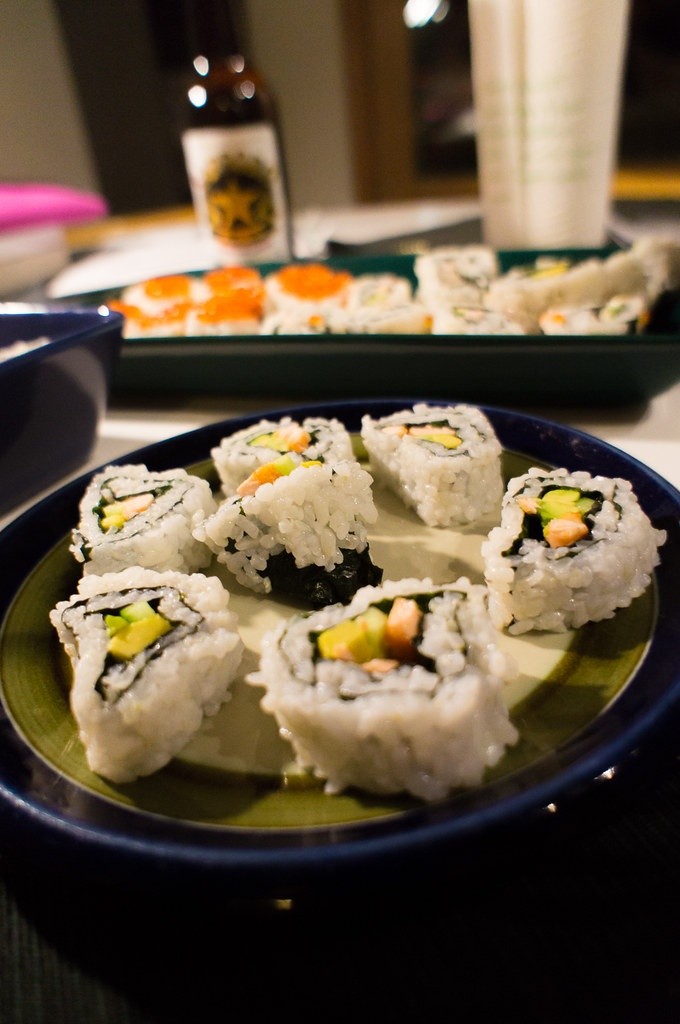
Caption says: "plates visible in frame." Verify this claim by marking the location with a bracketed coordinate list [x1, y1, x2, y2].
[0, 395, 680, 865]
[56, 247, 680, 424]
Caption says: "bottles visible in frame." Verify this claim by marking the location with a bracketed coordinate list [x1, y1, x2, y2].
[171, 0, 294, 266]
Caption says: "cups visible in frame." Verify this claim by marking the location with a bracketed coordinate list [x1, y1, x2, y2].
[469, 0, 629, 252]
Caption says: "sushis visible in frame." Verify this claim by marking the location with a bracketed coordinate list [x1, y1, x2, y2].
[112, 232, 668, 338]
[48, 402, 666, 799]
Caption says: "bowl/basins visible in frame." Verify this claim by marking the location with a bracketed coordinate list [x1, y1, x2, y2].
[0, 311, 121, 507]
